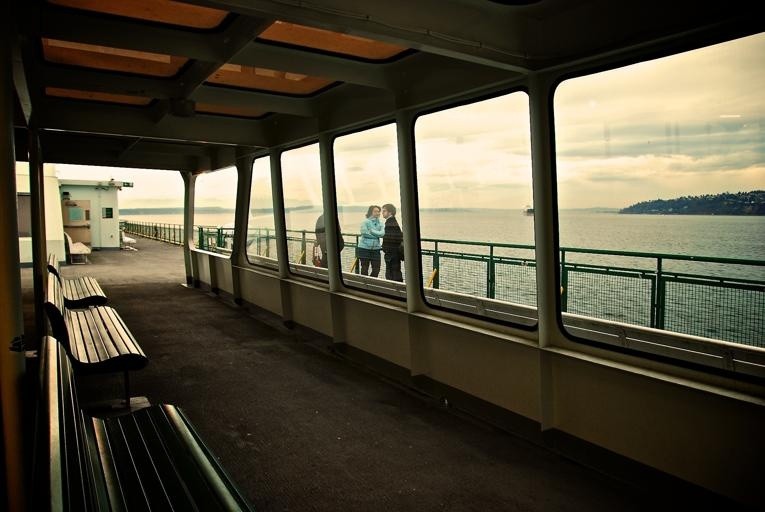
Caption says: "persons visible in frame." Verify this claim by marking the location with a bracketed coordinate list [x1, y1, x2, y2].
[315, 209, 345, 267]
[356, 205, 385, 277]
[381, 203, 404, 282]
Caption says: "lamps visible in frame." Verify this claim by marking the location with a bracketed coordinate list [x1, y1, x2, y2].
[95, 178, 116, 191]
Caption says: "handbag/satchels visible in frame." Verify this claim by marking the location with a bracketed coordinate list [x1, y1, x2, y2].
[391, 230, 404, 262]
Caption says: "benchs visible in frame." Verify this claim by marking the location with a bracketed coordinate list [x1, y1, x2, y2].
[39, 334, 256, 512]
[64, 232, 91, 264]
[45, 272, 152, 418]
[48, 253, 108, 307]
[120, 230, 137, 251]
